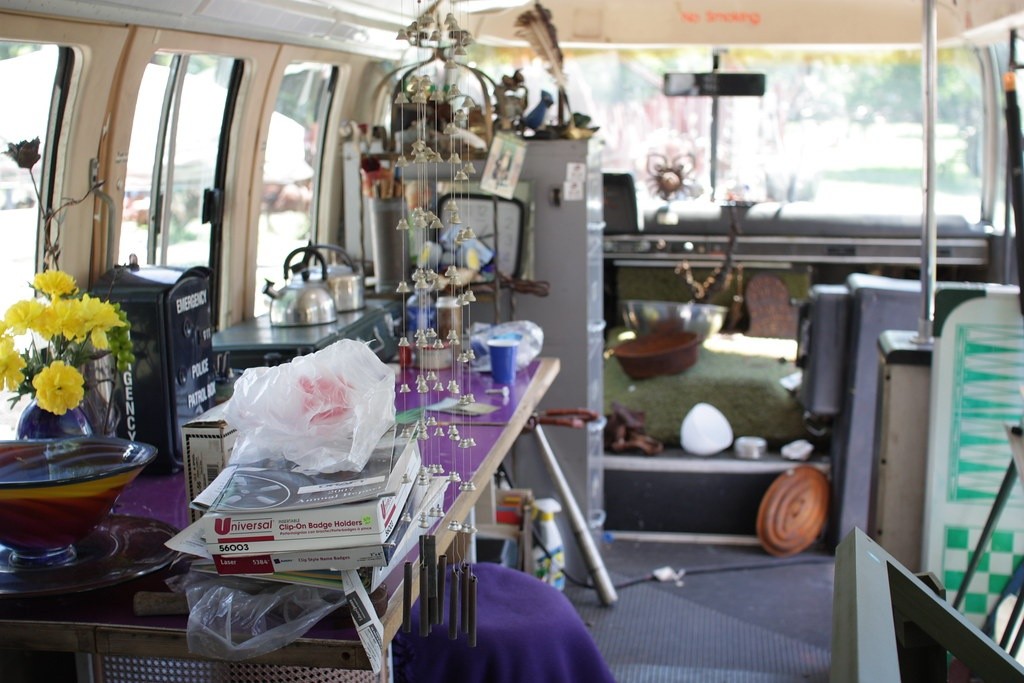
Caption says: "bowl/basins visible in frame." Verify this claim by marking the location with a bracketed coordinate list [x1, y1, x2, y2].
[734, 436, 767, 460]
[622, 299, 727, 344]
[680, 403, 733, 456]
[0, 439, 156, 566]
[613, 333, 699, 378]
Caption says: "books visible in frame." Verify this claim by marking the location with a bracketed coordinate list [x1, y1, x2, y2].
[189, 420, 421, 515]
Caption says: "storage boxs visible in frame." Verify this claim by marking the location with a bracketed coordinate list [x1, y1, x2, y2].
[207, 437, 424, 549]
[212, 466, 432, 576]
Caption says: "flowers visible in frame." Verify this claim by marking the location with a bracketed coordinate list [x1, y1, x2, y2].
[0, 266, 138, 420]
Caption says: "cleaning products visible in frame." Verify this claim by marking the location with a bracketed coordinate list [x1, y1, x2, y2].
[531, 499, 565, 591]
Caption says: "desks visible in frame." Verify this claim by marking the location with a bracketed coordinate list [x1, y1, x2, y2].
[0, 354, 619, 683]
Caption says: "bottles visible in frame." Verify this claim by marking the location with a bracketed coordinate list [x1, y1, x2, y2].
[406, 288, 434, 360]
[435, 284, 461, 359]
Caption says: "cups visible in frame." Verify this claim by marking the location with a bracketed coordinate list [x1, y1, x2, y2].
[487, 340, 519, 384]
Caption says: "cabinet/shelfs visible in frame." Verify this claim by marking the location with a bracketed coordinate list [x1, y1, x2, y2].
[216, 298, 405, 364]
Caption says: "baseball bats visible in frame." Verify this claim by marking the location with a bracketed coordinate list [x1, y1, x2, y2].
[534, 424, 619, 607]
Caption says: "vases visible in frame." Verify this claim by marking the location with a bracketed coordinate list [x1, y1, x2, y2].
[16, 396, 95, 440]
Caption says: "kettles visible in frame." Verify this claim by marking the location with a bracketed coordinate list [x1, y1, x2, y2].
[291, 245, 364, 312]
[262, 247, 336, 325]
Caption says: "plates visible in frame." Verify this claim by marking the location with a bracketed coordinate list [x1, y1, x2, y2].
[0, 515, 180, 598]
